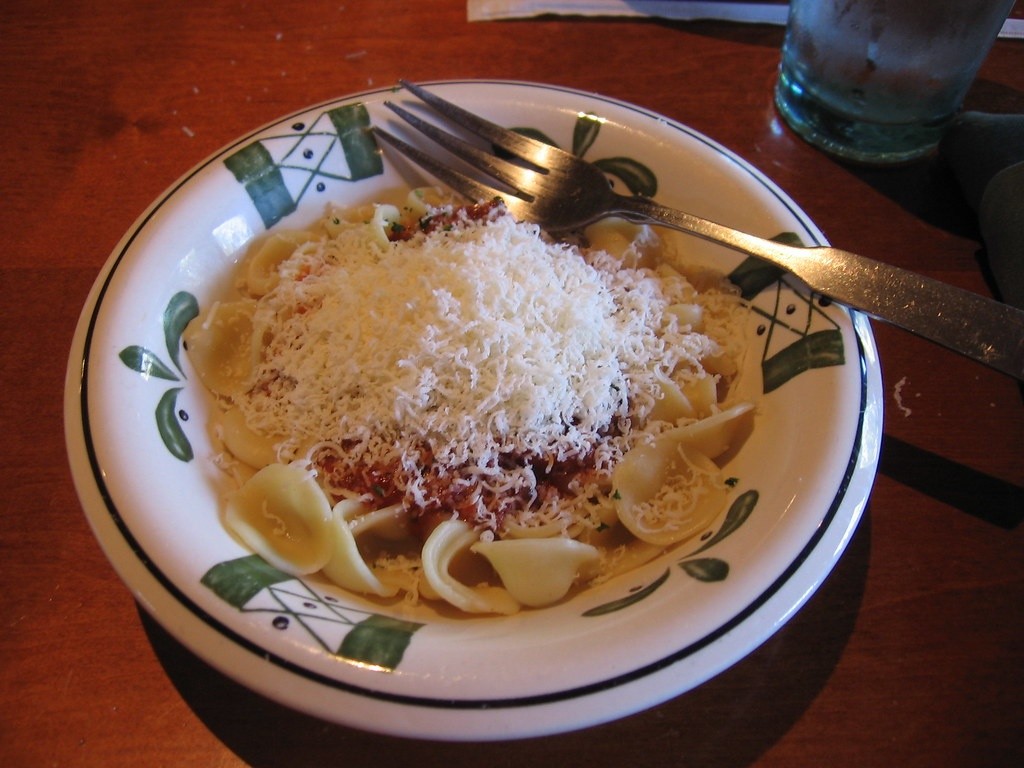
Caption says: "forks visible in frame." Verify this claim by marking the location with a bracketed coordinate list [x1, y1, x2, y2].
[368, 80, 1024, 384]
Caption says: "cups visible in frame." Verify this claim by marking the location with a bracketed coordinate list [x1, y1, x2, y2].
[775, 1, 1017, 167]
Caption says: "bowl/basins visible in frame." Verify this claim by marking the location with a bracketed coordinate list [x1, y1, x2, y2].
[62, 77, 884, 744]
